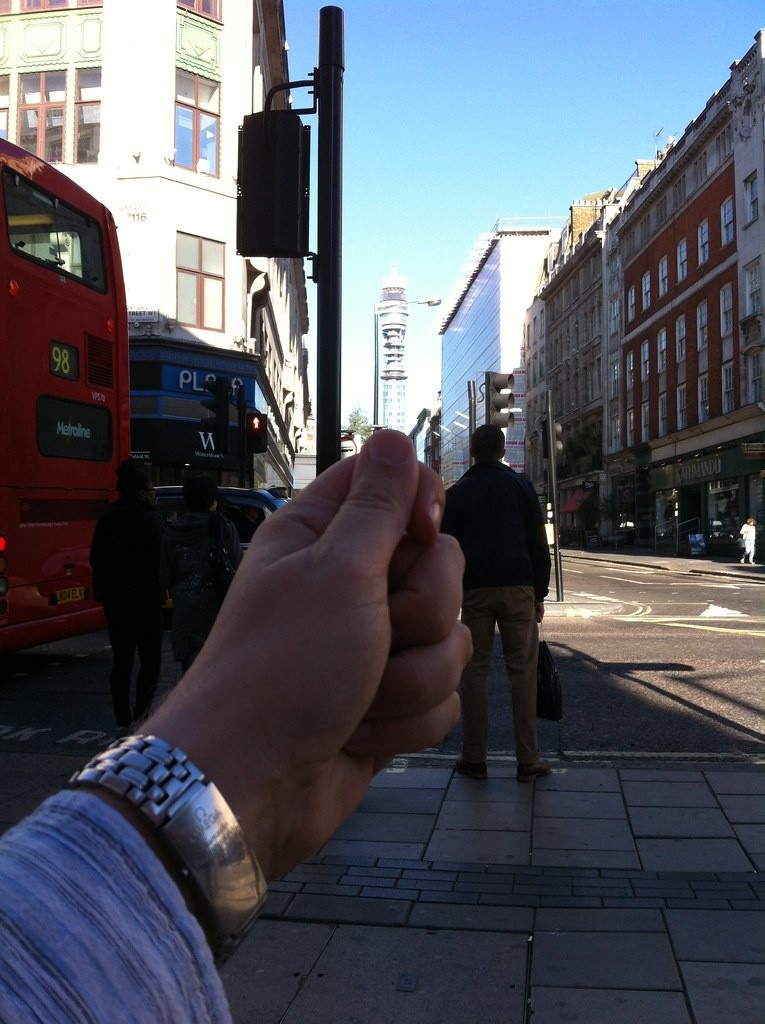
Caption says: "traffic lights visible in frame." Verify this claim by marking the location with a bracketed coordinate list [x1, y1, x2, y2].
[488, 371, 515, 430]
[245, 413, 267, 436]
[552, 419, 563, 455]
[197, 376, 225, 455]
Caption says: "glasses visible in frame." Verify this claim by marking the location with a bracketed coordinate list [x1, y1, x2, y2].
[143, 488, 156, 494]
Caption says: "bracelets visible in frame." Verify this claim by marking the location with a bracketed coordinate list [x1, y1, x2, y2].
[67, 735, 268, 972]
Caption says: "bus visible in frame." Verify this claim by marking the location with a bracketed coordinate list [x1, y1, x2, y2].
[0, 139, 130, 656]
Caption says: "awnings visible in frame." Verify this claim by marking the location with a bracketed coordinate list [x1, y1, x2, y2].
[560, 486, 594, 513]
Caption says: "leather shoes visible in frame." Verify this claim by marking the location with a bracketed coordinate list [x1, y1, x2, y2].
[516, 761, 551, 783]
[456, 758, 487, 779]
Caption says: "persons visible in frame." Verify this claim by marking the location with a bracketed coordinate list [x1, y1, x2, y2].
[439, 425, 552, 783]
[741, 518, 757, 564]
[663, 501, 674, 530]
[90, 459, 259, 737]
[0, 428, 473, 1024]
[715, 490, 739, 529]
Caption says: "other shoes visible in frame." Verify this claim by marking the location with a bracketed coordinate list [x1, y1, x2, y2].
[117, 719, 135, 731]
[132, 707, 149, 723]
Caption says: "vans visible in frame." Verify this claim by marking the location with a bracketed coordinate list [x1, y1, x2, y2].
[149, 485, 288, 561]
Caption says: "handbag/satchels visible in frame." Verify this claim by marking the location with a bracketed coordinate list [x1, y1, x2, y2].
[211, 514, 235, 597]
[535, 640, 564, 722]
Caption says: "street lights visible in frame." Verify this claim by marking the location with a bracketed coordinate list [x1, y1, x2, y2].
[371, 295, 442, 435]
